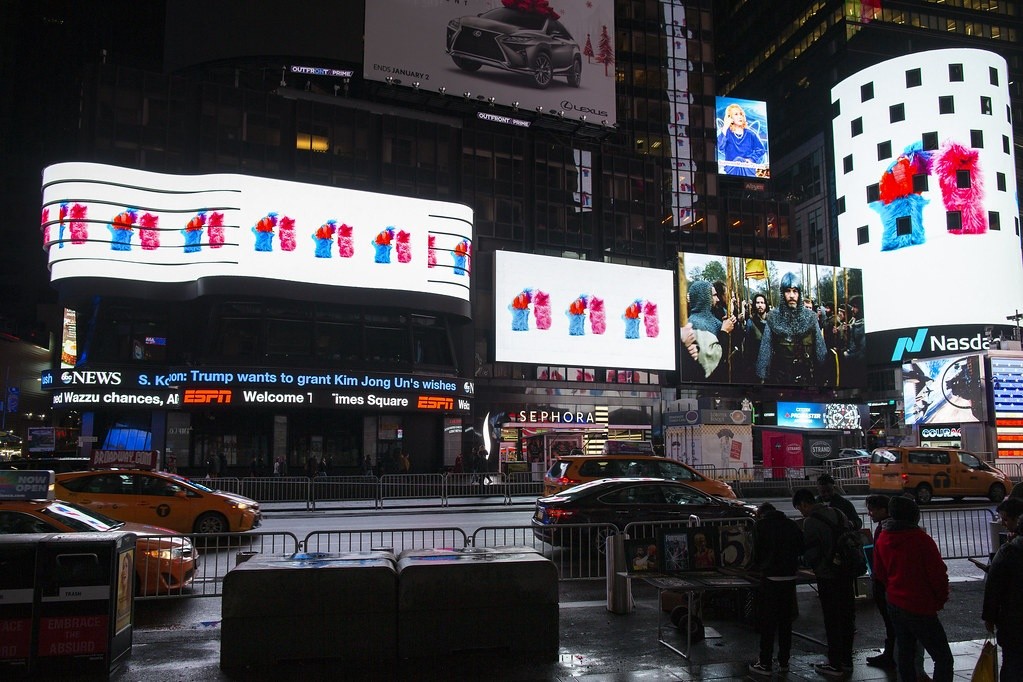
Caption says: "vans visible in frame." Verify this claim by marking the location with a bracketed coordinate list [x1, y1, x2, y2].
[868, 447, 1011, 506]
[544, 455, 737, 501]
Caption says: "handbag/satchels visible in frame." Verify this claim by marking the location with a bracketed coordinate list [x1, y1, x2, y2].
[972, 632, 998, 682]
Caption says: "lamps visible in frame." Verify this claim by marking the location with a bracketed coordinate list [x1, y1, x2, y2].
[601, 120, 608, 127]
[558, 110, 565, 120]
[385, 76, 393, 88]
[580, 115, 587, 124]
[536, 106, 543, 116]
[412, 81, 420, 93]
[438, 86, 446, 97]
[343, 78, 349, 98]
[512, 101, 519, 112]
[488, 97, 496, 109]
[463, 92, 471, 103]
[989, 377, 1001, 390]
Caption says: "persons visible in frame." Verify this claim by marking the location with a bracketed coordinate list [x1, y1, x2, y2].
[717, 105, 765, 177]
[815, 470, 863, 632]
[360, 454, 409, 476]
[680, 280, 768, 382]
[121, 553, 132, 610]
[719, 436, 731, 478]
[866, 495, 894, 666]
[306, 455, 335, 475]
[975, 481, 1023, 682]
[801, 294, 866, 386]
[273, 455, 287, 477]
[873, 497, 954, 682]
[746, 503, 799, 674]
[757, 271, 837, 385]
[792, 488, 854, 676]
[633, 546, 657, 570]
[455, 445, 493, 483]
[250, 456, 264, 480]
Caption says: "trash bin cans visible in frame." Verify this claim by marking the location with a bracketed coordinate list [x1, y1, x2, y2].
[990, 521, 1009, 553]
[833, 466, 854, 477]
[606, 534, 631, 612]
[0, 531, 138, 678]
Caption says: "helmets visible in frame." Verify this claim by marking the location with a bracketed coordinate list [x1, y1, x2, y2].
[780, 274, 806, 315]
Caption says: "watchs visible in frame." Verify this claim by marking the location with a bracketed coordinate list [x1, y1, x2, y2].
[823, 404, 862, 430]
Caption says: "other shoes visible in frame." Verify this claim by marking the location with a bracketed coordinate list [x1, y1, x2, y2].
[866, 655, 897, 667]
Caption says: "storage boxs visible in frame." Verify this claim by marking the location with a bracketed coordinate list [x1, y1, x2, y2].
[660, 590, 683, 612]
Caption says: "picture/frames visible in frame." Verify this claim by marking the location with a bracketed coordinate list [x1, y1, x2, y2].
[655, 527, 688, 572]
[623, 538, 658, 575]
[687, 526, 721, 572]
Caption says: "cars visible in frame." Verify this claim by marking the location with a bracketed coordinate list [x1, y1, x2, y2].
[54, 471, 264, 549]
[445, 6, 582, 90]
[0, 470, 200, 596]
[839, 448, 869, 462]
[531, 478, 760, 560]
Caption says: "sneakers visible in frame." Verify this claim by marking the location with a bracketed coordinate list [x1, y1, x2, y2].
[841, 663, 854, 672]
[813, 664, 844, 676]
[772, 657, 788, 671]
[749, 662, 771, 675]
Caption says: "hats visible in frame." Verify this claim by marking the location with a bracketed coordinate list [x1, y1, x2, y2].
[887, 495, 921, 522]
[849, 295, 863, 309]
[838, 304, 853, 315]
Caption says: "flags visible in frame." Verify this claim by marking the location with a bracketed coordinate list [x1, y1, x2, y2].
[745, 259, 768, 280]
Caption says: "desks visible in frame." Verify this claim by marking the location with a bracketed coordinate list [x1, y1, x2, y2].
[618, 571, 819, 660]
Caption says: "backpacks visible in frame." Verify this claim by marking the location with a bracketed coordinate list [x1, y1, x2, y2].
[811, 508, 867, 572]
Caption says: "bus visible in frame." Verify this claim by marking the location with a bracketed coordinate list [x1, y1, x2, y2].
[27, 427, 80, 455]
